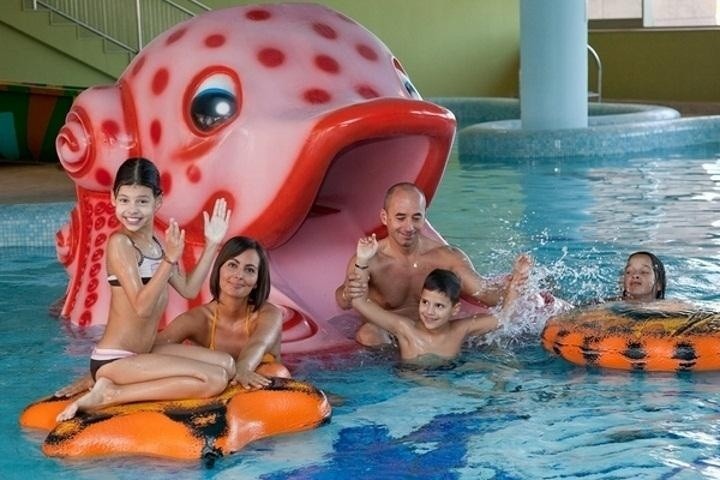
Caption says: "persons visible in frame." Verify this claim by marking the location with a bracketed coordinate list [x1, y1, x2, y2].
[334, 181, 529, 362]
[52, 156, 238, 425]
[53, 235, 292, 400]
[350, 232, 534, 414]
[587, 251, 667, 305]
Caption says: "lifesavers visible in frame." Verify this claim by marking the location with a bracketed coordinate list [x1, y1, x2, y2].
[20, 361, 332, 467]
[541, 300, 719, 373]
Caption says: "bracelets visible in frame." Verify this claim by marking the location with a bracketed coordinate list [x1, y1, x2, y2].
[163, 256, 177, 265]
[355, 263, 368, 269]
